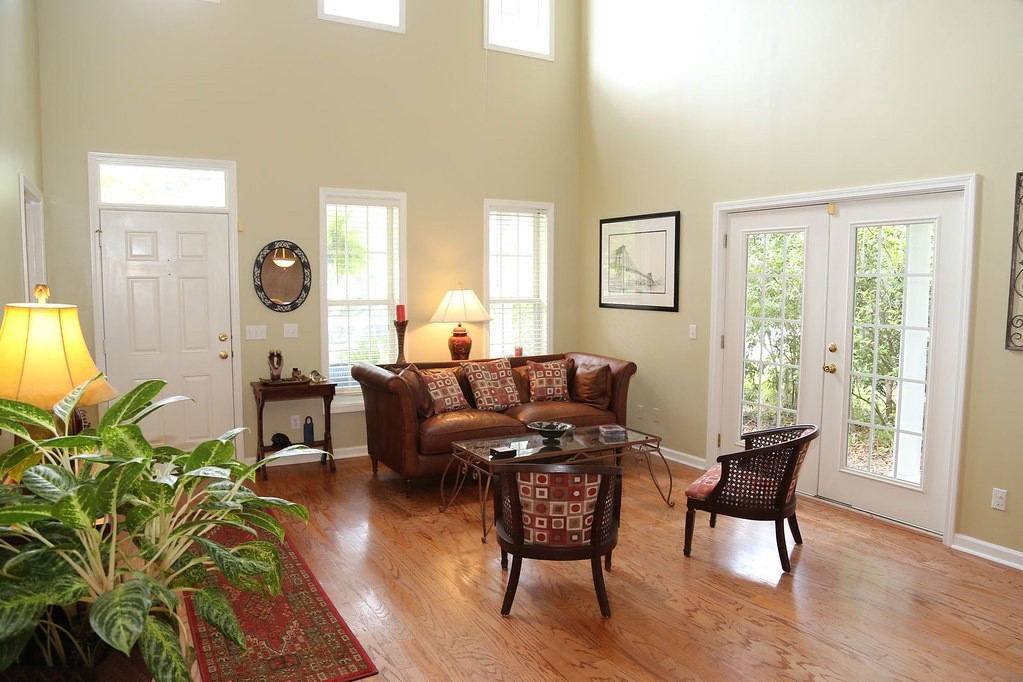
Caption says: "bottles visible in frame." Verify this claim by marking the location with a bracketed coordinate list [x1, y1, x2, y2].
[304, 416, 314, 447]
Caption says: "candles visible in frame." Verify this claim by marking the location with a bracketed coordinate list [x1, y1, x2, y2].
[395, 305, 405, 322]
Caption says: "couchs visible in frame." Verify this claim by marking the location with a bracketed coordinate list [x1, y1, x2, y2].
[351, 351, 638, 498]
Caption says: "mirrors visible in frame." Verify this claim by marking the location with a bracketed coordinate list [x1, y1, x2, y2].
[253, 241, 311, 312]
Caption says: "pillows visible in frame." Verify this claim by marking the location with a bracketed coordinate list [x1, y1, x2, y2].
[527, 359, 572, 403]
[571, 362, 612, 411]
[418, 367, 472, 414]
[459, 357, 522, 411]
[399, 362, 433, 425]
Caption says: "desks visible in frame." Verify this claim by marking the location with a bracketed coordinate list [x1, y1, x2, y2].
[250, 379, 338, 482]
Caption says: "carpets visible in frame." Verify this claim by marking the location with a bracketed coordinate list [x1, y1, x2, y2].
[166, 506, 379, 682]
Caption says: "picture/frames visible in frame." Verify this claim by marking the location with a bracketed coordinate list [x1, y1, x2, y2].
[598, 210, 681, 312]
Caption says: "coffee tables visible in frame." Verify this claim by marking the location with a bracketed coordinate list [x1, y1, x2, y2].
[438, 421, 674, 541]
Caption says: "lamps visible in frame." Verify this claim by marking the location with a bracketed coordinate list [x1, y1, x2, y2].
[429, 282, 493, 361]
[270, 247, 297, 267]
[0, 283, 119, 468]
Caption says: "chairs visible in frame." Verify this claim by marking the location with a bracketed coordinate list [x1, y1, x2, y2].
[683, 424, 820, 570]
[488, 462, 625, 618]
